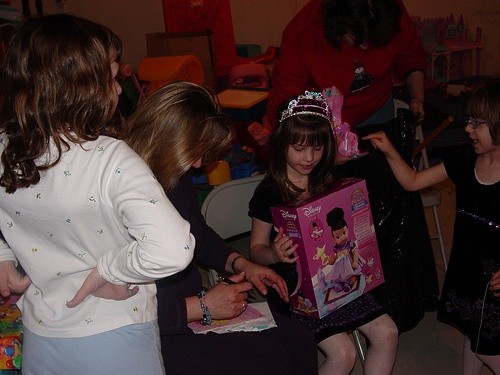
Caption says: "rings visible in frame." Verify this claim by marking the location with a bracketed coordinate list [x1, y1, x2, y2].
[241, 302, 245, 310]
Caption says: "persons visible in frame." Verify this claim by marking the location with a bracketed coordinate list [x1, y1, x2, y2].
[266, 0, 426, 134]
[363, 82, 500, 375]
[118, 82, 318, 374]
[248, 91, 399, 375]
[0, 12, 195, 375]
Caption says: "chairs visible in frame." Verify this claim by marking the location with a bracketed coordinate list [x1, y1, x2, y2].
[202, 175, 367, 366]
[394, 99, 447, 274]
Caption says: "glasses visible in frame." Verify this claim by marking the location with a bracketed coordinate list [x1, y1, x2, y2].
[466, 118, 489, 129]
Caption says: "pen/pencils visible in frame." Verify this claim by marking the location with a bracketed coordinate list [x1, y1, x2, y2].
[217, 273, 256, 299]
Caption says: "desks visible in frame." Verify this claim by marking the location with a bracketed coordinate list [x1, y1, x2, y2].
[428, 39, 484, 83]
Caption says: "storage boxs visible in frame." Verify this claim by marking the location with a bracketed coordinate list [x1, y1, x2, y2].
[270, 179, 385, 318]
[147, 32, 214, 69]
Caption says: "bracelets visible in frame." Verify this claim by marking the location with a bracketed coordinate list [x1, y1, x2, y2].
[232, 256, 245, 274]
[201, 290, 211, 325]
[197, 292, 206, 325]
